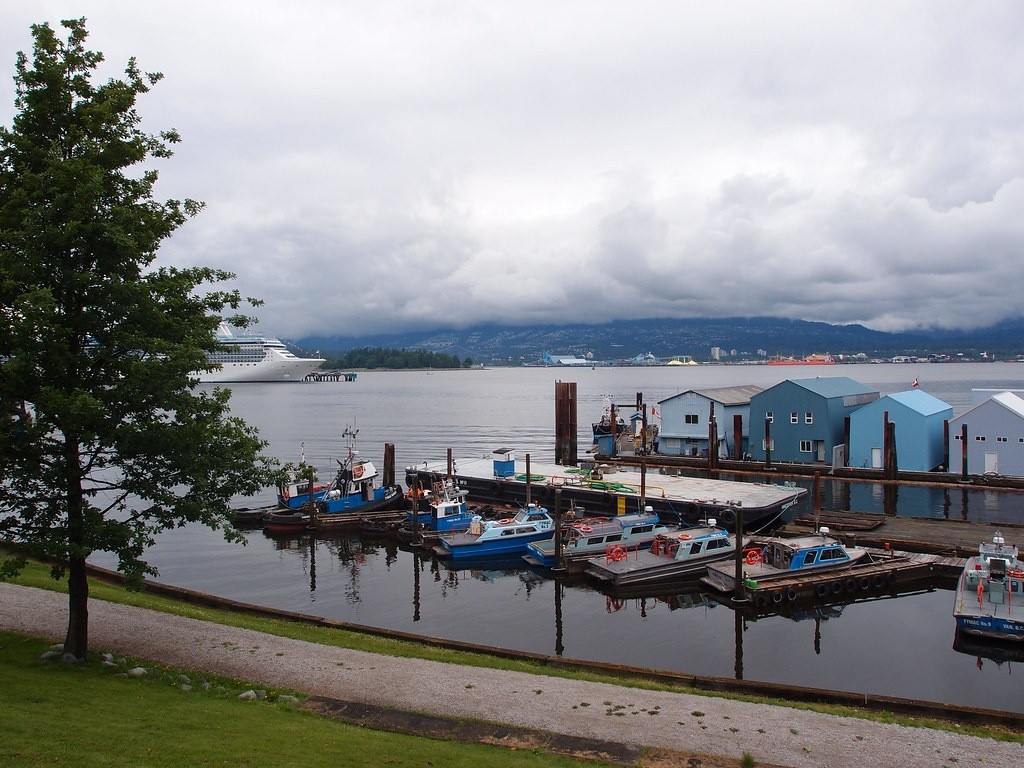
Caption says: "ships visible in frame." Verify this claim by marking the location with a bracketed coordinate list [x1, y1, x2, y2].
[82, 320, 328, 384]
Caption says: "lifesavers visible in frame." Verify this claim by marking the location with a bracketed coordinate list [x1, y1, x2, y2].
[498, 519, 510, 524]
[612, 547, 623, 561]
[354, 466, 363, 477]
[573, 524, 586, 527]
[581, 526, 593, 533]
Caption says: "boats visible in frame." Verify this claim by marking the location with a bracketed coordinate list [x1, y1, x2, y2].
[228, 467, 332, 525]
[952, 623, 1024, 666]
[357, 475, 481, 542]
[262, 459, 404, 531]
[520, 506, 681, 571]
[953, 529, 1024, 639]
[431, 503, 586, 559]
[584, 519, 751, 585]
[699, 526, 867, 593]
[427, 558, 849, 622]
